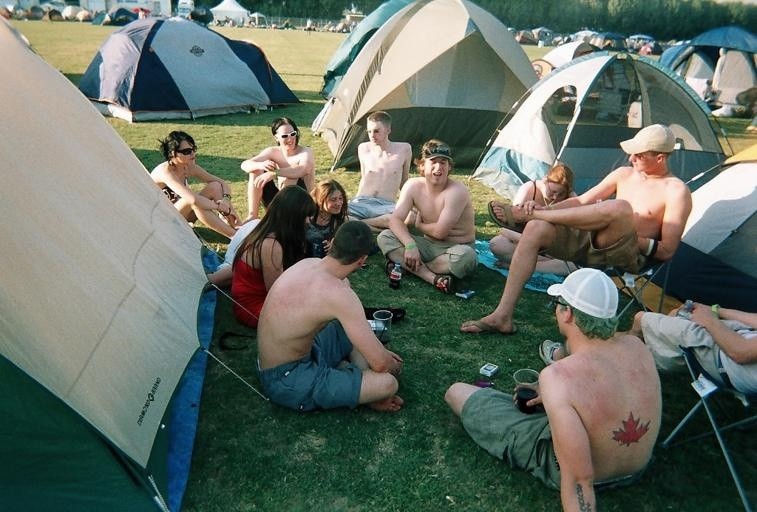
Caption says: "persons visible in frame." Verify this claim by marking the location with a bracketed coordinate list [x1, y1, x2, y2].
[240, 117, 315, 224]
[231, 184, 315, 328]
[346, 111, 413, 231]
[205, 180, 348, 328]
[444, 268, 663, 512]
[149, 130, 242, 239]
[257, 221, 404, 413]
[539, 301, 757, 395]
[376, 139, 477, 294]
[459, 122, 693, 335]
[488, 165, 585, 275]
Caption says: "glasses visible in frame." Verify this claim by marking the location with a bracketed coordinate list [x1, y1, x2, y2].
[274, 131, 298, 139]
[175, 146, 197, 156]
[553, 301, 567, 308]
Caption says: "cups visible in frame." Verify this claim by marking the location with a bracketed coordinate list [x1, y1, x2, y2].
[373, 310, 393, 345]
[513, 369, 540, 415]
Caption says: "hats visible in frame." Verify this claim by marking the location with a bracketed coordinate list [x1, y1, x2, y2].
[422, 155, 454, 162]
[620, 123, 675, 155]
[547, 267, 619, 318]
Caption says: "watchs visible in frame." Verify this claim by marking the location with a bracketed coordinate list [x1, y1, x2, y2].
[223, 193, 231, 199]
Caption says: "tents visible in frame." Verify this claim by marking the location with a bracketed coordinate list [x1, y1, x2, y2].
[317, 0, 426, 100]
[605, 142, 756, 317]
[658, 25, 757, 116]
[77, 18, 305, 121]
[468, 52, 735, 204]
[311, 1, 539, 173]
[0, 17, 269, 512]
[205, 0, 250, 27]
[540, 41, 602, 70]
[513, 26, 666, 56]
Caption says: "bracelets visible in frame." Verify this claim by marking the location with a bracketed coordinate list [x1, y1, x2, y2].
[642, 238, 654, 257]
[274, 169, 279, 178]
[646, 240, 657, 257]
[405, 241, 417, 249]
[711, 304, 720, 318]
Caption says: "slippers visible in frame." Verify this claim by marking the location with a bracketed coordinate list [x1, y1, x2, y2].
[488, 200, 529, 235]
[539, 338, 563, 367]
[461, 319, 519, 335]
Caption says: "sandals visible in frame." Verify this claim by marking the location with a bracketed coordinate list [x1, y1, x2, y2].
[434, 274, 452, 294]
[385, 259, 405, 276]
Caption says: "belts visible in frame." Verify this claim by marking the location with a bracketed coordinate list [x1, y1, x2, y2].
[719, 360, 738, 393]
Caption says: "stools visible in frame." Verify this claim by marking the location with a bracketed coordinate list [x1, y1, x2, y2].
[662, 344, 757, 512]
[562, 259, 670, 328]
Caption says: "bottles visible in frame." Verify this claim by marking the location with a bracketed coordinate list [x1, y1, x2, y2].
[388, 264, 401, 289]
